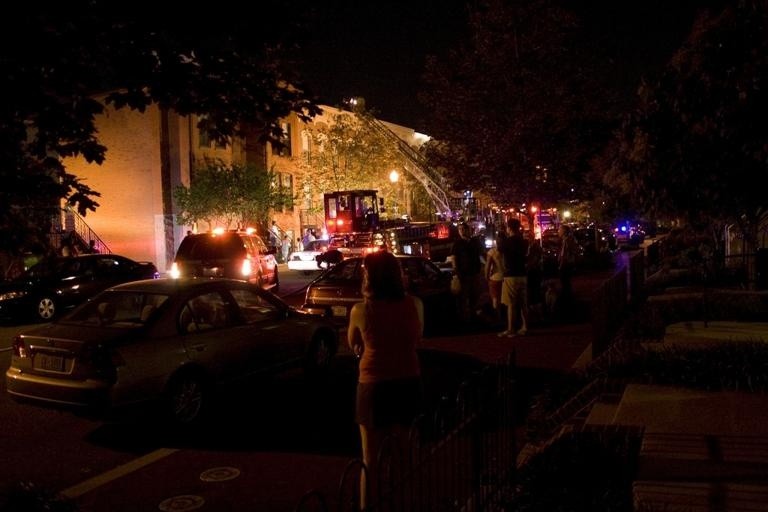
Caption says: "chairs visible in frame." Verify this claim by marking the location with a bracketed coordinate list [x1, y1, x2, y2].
[98, 303, 117, 320]
[141, 305, 157, 320]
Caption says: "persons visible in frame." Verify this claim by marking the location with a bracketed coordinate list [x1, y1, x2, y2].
[83, 240, 99, 254]
[270, 219, 317, 265]
[450, 217, 575, 339]
[364, 207, 377, 232]
[187, 230, 192, 234]
[347, 248, 427, 512]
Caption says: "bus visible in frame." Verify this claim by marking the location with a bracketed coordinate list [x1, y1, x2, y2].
[534, 214, 559, 241]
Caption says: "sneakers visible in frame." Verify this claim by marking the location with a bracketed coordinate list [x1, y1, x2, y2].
[498, 329, 527, 337]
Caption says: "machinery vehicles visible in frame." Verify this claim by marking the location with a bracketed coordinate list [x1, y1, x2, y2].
[313, 190, 486, 272]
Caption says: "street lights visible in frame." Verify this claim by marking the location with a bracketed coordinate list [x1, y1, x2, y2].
[390, 170, 399, 220]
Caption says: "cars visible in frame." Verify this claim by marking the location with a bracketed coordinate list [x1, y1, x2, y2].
[0, 254, 157, 322]
[304, 255, 451, 349]
[5, 275, 341, 425]
[171, 232, 283, 295]
